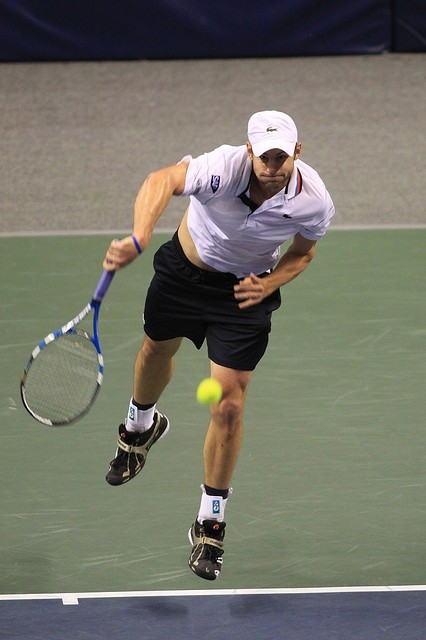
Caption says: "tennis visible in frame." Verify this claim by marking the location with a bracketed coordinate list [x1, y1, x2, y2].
[196, 378, 222, 405]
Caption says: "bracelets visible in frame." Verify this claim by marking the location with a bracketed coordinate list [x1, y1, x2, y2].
[130, 235, 142, 254]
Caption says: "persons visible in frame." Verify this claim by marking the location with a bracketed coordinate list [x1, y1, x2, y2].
[101, 109, 335, 581]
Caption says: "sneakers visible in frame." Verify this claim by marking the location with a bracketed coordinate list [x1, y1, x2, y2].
[106, 410, 170, 485]
[187, 516, 226, 579]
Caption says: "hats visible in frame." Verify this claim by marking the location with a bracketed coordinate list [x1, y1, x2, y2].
[248, 110, 297, 156]
